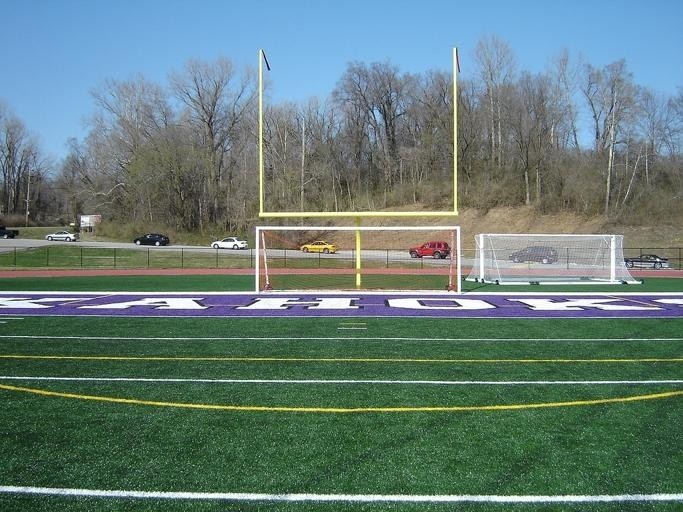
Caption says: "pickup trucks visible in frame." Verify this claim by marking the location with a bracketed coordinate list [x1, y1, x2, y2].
[0, 225, 20, 239]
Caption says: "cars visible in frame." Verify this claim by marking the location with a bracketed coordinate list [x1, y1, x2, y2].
[620, 254, 669, 270]
[45, 230, 80, 241]
[133, 234, 170, 246]
[210, 236, 249, 250]
[300, 240, 337, 254]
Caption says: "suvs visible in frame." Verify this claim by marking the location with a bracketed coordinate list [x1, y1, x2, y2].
[509, 246, 558, 264]
[409, 241, 451, 260]
